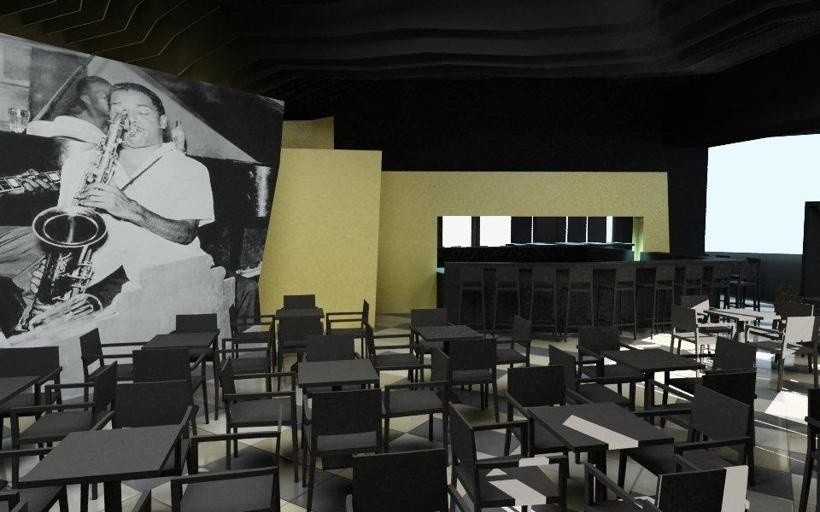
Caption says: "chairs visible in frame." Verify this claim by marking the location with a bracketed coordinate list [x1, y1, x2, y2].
[437, 251, 766, 341]
[0, 294, 819, 512]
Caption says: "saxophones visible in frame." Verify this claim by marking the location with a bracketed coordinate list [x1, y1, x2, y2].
[20, 109, 141, 330]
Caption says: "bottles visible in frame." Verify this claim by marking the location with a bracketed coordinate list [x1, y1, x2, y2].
[173, 121, 186, 156]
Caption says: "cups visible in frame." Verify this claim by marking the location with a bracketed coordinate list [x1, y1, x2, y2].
[8, 106, 31, 134]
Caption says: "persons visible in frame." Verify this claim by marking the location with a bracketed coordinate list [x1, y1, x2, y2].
[64, 76, 112, 146]
[0, 83, 261, 351]
